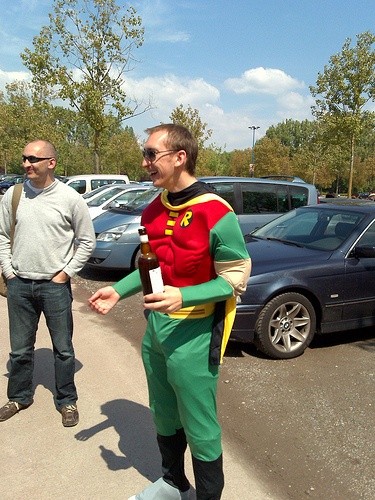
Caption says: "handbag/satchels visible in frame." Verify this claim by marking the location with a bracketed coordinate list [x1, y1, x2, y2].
[0, 183, 23, 298]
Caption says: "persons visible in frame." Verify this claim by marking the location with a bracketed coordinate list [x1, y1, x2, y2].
[89, 125, 252, 500]
[0, 140, 96, 427]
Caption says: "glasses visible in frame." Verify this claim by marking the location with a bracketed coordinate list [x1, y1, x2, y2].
[21, 155, 53, 163]
[141, 148, 179, 162]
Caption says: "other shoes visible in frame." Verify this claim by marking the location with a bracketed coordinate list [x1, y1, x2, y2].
[62, 403, 79, 427]
[1, 401, 23, 421]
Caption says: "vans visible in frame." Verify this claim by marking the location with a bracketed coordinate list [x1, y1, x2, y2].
[84, 172, 319, 273]
[64, 174, 130, 197]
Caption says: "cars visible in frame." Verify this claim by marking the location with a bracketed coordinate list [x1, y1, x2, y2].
[229, 202, 375, 360]
[83, 183, 152, 221]
[0, 174, 25, 194]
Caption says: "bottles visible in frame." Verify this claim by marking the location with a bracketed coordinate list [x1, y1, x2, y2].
[138, 226, 164, 303]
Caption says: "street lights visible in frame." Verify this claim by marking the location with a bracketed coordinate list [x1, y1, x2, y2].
[248, 125, 260, 177]
